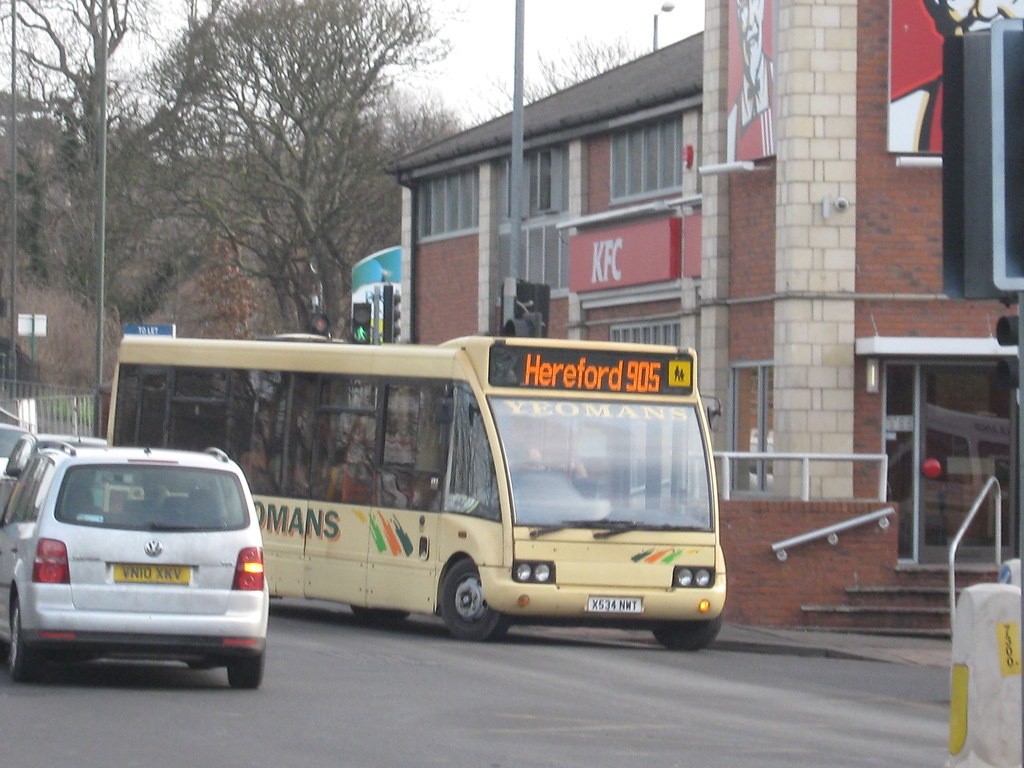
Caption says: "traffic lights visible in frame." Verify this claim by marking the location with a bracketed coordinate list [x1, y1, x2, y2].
[311, 313, 330, 334]
[921, 454, 947, 482]
[987, 314, 1020, 421]
[504, 311, 544, 338]
[383, 284, 401, 343]
[352, 303, 371, 345]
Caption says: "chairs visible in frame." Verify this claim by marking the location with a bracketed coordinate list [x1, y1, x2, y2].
[64, 482, 113, 521]
[183, 490, 222, 527]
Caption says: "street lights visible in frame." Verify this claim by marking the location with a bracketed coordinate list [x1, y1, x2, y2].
[652, 0, 675, 52]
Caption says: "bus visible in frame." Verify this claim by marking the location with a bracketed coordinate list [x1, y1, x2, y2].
[106, 333, 728, 653]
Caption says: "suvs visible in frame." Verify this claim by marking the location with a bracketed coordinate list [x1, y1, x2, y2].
[0, 422, 104, 521]
[0, 440, 270, 689]
